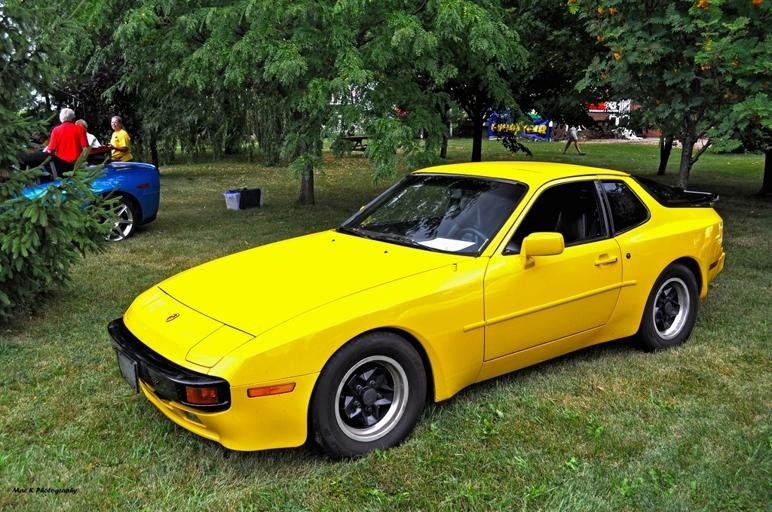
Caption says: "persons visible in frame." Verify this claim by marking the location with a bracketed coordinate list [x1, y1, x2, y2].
[41, 107, 88, 192]
[562, 123, 586, 156]
[1, 130, 49, 182]
[104, 116, 134, 165]
[73, 118, 104, 150]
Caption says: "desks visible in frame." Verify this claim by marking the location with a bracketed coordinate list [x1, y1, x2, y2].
[341, 136, 368, 151]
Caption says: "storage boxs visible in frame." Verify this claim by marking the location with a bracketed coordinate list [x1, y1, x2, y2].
[222, 188, 265, 211]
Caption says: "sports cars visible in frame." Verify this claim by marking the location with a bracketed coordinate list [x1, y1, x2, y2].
[107, 154, 727, 463]
[0, 152, 160, 243]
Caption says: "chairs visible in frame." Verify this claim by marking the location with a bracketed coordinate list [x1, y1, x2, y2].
[435, 186, 595, 254]
